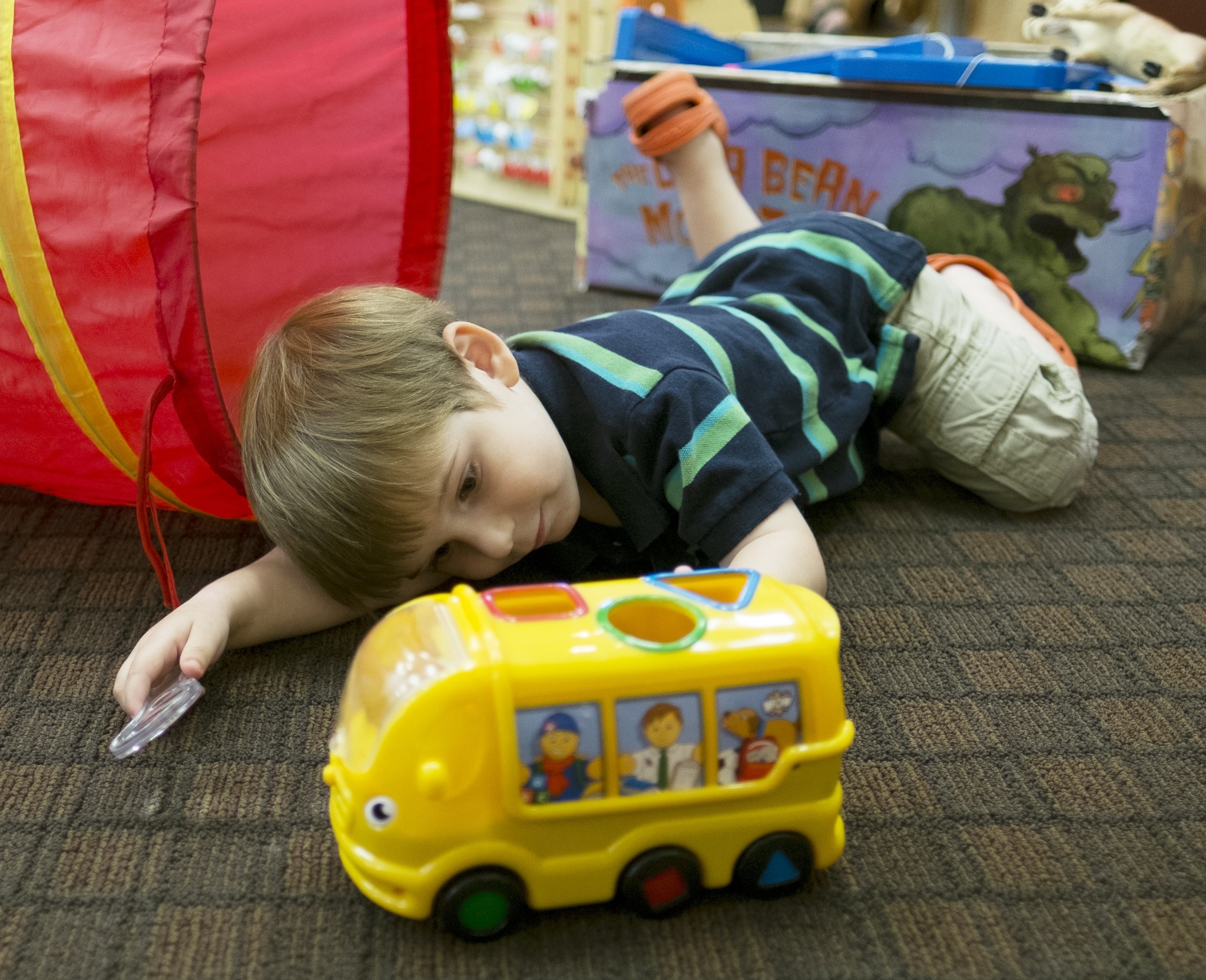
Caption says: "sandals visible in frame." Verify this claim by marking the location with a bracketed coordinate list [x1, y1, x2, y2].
[924, 253, 1079, 371]
[621, 69, 727, 163]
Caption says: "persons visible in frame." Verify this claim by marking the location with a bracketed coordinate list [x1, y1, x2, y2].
[112, 66, 1099, 718]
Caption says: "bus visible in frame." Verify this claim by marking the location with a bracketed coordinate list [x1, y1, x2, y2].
[323, 565, 860, 944]
